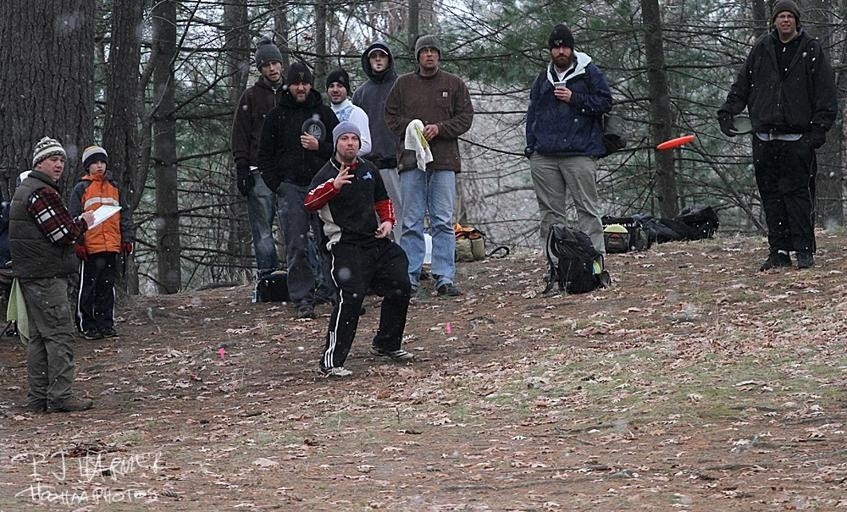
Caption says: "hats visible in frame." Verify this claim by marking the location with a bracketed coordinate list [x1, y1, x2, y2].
[368, 48, 388, 58]
[549, 24, 573, 52]
[82, 146, 109, 170]
[327, 68, 349, 95]
[771, 0, 800, 25]
[31, 137, 67, 168]
[332, 121, 361, 152]
[254, 40, 283, 69]
[414, 34, 441, 61]
[287, 62, 311, 84]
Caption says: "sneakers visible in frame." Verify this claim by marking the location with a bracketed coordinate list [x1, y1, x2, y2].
[333, 299, 364, 315]
[79, 328, 103, 340]
[760, 250, 792, 272]
[294, 300, 315, 319]
[796, 252, 813, 267]
[433, 276, 458, 296]
[47, 397, 92, 413]
[100, 327, 116, 338]
[317, 366, 352, 377]
[369, 344, 414, 360]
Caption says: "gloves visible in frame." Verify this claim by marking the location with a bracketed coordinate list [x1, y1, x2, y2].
[718, 112, 738, 137]
[237, 175, 254, 196]
[122, 242, 132, 257]
[72, 245, 88, 262]
[808, 126, 826, 148]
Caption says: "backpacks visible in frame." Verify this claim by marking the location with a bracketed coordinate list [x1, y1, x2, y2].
[601, 204, 719, 253]
[542, 223, 611, 294]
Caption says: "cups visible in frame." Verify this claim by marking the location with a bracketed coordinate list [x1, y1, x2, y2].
[553, 82, 567, 98]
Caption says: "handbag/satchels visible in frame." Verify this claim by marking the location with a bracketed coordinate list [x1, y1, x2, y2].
[602, 113, 627, 153]
[454, 229, 485, 262]
[256, 269, 289, 302]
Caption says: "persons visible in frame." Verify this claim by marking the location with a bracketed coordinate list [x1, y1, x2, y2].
[231, 34, 473, 378]
[717, 0, 838, 272]
[9, 135, 96, 414]
[524, 24, 627, 282]
[68, 145, 135, 340]
